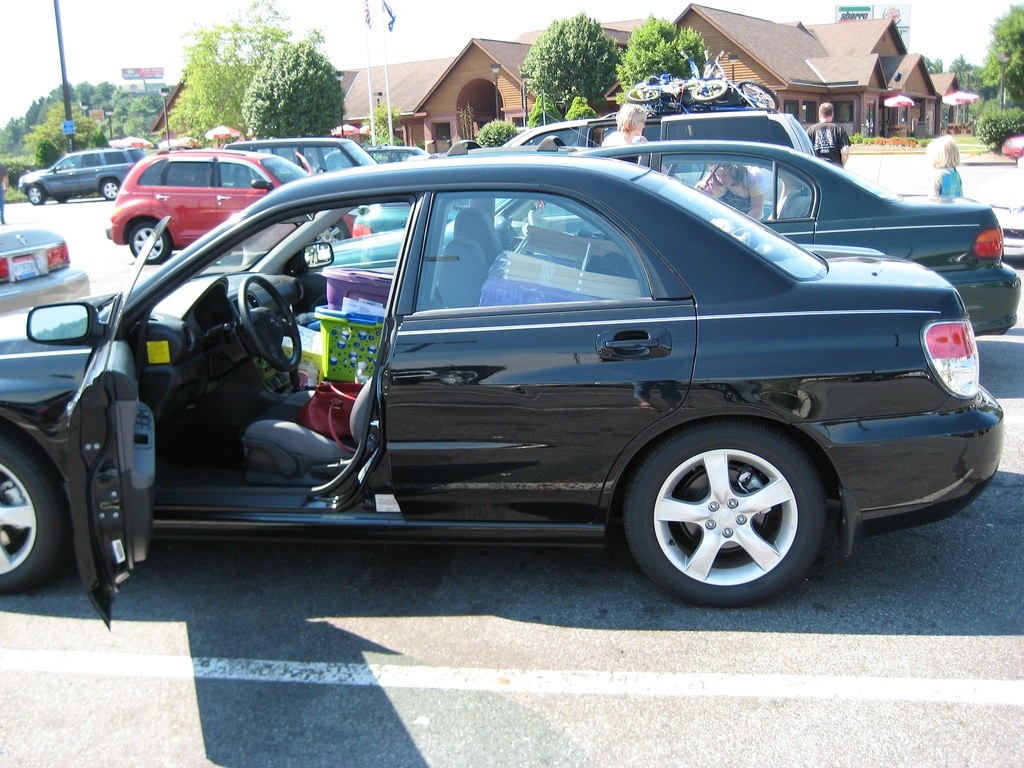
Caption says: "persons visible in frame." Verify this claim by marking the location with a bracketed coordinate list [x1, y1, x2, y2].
[0, 163, 9, 224]
[695, 162, 766, 221]
[600, 102, 678, 181]
[926, 135, 964, 197]
[806, 103, 851, 168]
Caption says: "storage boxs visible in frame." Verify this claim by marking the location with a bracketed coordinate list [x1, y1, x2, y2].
[258, 266, 393, 386]
[477, 251, 605, 307]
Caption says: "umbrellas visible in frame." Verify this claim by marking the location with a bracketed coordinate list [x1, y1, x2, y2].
[158, 136, 197, 150]
[331, 123, 358, 139]
[883, 95, 914, 126]
[107, 136, 155, 150]
[205, 126, 240, 144]
[942, 91, 979, 124]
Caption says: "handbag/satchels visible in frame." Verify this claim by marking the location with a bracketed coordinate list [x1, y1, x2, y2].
[297, 381, 365, 455]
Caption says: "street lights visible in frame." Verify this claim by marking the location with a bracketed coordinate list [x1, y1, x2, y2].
[727, 54, 739, 85]
[490, 63, 501, 121]
[373, 91, 383, 107]
[104, 108, 114, 140]
[335, 71, 344, 138]
[996, 50, 1012, 111]
[159, 87, 170, 147]
[79, 101, 90, 118]
[520, 73, 530, 127]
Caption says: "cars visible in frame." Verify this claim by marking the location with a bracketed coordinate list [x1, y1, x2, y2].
[317, 144, 430, 174]
[307, 136, 1021, 338]
[0, 223, 90, 317]
[0, 151, 1005, 630]
[1001, 135, 1024, 162]
[105, 149, 362, 265]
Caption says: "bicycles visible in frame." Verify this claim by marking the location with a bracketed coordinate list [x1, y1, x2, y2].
[625, 50, 780, 114]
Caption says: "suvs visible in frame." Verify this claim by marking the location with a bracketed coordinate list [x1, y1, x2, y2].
[225, 137, 378, 176]
[18, 147, 150, 205]
[492, 108, 818, 213]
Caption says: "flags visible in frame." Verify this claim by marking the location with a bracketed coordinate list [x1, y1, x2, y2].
[383, 0, 396, 31]
[365, 0, 371, 29]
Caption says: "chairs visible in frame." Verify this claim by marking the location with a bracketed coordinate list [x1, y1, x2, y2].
[392, 155, 398, 162]
[240, 237, 488, 485]
[453, 206, 503, 259]
[776, 189, 802, 219]
[788, 195, 810, 218]
[396, 153, 402, 162]
[231, 166, 253, 188]
